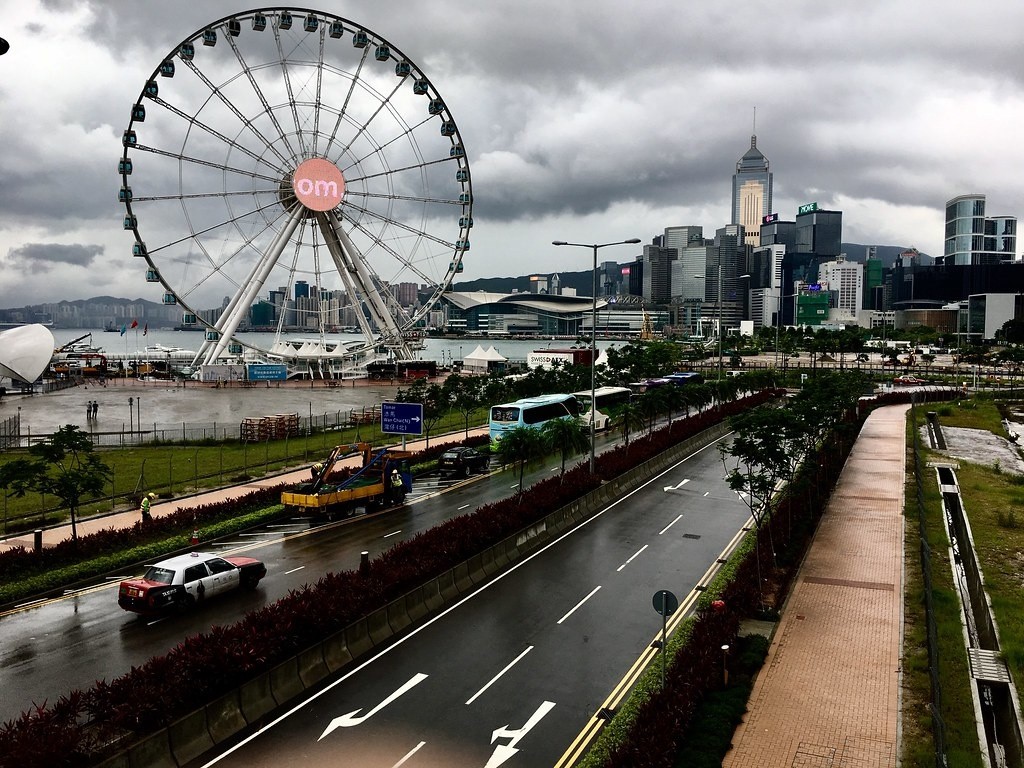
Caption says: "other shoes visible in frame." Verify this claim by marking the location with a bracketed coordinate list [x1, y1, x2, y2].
[400, 503, 403, 506]
[395, 505, 400, 506]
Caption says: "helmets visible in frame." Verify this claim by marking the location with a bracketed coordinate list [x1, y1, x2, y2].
[148, 492, 156, 500]
[392, 470, 397, 473]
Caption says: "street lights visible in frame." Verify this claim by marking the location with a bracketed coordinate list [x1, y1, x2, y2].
[693, 274, 750, 415]
[758, 293, 800, 374]
[551, 237, 641, 478]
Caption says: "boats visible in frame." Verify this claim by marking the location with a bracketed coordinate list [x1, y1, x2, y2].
[54, 331, 103, 354]
[145, 343, 184, 353]
[102, 320, 121, 333]
[373, 329, 430, 353]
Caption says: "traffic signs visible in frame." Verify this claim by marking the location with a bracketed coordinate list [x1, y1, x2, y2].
[381, 401, 424, 437]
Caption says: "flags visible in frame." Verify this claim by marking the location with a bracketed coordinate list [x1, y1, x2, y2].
[142, 324, 148, 337]
[130, 320, 138, 329]
[119, 323, 127, 337]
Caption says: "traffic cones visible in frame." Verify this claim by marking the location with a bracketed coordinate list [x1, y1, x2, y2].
[190, 527, 199, 546]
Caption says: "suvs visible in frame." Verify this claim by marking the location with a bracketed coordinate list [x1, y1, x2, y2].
[437, 447, 491, 479]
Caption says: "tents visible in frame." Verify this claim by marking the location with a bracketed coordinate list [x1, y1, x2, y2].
[464, 345, 507, 373]
[267, 341, 350, 367]
[241, 343, 264, 364]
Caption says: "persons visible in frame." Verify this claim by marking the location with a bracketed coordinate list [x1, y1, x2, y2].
[140, 493, 156, 522]
[390, 470, 404, 508]
[311, 462, 328, 484]
[85, 400, 100, 421]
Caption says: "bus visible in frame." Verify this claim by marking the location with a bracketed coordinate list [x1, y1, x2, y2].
[663, 372, 703, 386]
[629, 378, 671, 401]
[489, 394, 586, 452]
[569, 386, 632, 436]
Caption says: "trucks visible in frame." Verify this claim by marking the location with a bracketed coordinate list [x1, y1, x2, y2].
[281, 440, 416, 524]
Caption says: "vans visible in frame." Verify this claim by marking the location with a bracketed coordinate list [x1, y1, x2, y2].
[726, 369, 749, 379]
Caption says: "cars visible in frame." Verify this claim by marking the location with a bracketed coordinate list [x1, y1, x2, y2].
[893, 374, 930, 386]
[118, 552, 267, 620]
